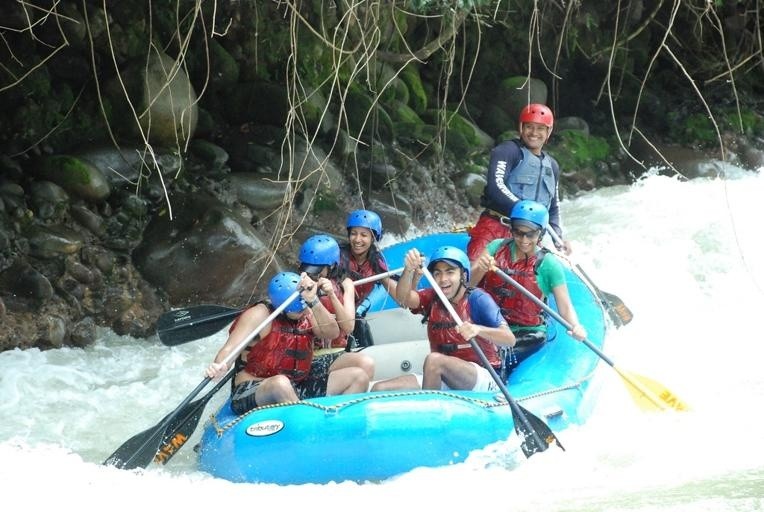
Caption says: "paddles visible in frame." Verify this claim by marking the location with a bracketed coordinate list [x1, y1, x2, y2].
[419, 262, 567, 458]
[491, 263, 689, 416]
[545, 221, 634, 331]
[103, 288, 301, 473]
[157, 267, 404, 346]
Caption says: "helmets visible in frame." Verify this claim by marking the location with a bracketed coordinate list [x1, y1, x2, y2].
[345, 209, 382, 242]
[510, 199, 549, 234]
[268, 272, 312, 314]
[297, 234, 340, 269]
[427, 246, 471, 284]
[518, 103, 553, 142]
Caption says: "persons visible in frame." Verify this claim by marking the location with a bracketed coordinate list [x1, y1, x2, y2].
[336, 209, 425, 347]
[298, 234, 356, 348]
[469, 199, 587, 385]
[204, 270, 376, 413]
[467, 103, 572, 267]
[370, 246, 517, 393]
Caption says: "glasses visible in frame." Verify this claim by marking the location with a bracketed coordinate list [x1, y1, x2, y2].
[513, 227, 539, 238]
